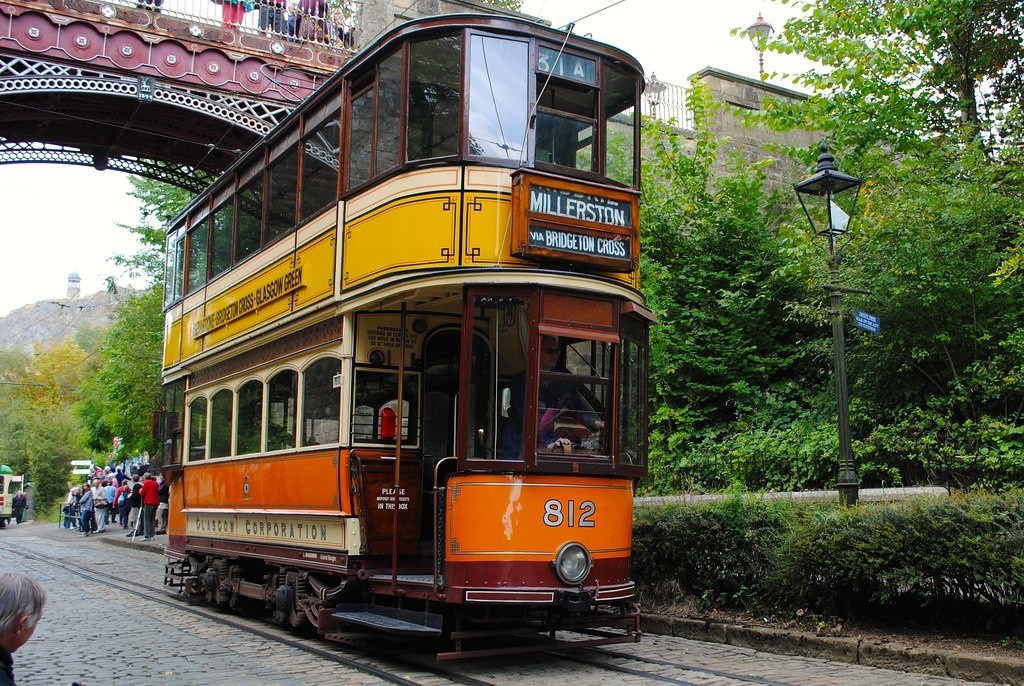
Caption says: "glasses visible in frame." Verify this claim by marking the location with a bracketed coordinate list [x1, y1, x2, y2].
[541, 348, 560, 355]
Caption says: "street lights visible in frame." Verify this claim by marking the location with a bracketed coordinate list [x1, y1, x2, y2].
[644, 72, 666, 119]
[792, 141, 867, 509]
[746, 12, 775, 76]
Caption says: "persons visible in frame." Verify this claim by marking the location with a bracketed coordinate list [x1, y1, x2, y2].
[135, 0, 165, 14]
[212, 0, 355, 52]
[157, 474, 169, 534]
[14, 489, 27, 524]
[91, 480, 109, 534]
[63, 452, 151, 537]
[138, 472, 159, 542]
[500, 333, 605, 460]
[127, 474, 143, 536]
[0, 571, 87, 686]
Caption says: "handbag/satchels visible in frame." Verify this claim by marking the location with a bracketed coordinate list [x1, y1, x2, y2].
[118, 492, 124, 505]
[95, 499, 109, 508]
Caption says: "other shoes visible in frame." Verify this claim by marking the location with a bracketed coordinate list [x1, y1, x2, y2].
[155, 528, 166, 534]
[78, 531, 88, 538]
[126, 529, 138, 536]
[93, 530, 105, 533]
[140, 536, 155, 541]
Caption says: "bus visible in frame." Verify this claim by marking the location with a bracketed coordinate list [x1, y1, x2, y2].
[151, 13, 660, 662]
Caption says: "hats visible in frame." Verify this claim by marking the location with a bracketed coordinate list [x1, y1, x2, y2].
[132, 474, 139, 480]
